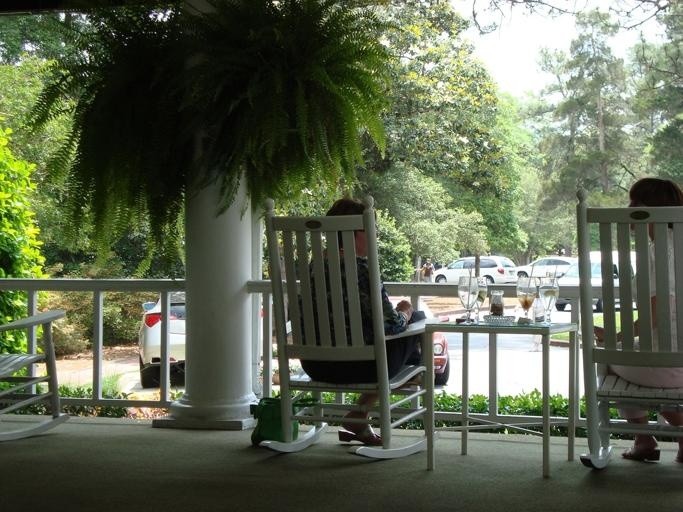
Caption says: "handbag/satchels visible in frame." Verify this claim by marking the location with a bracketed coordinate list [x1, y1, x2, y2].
[250, 399, 298, 446]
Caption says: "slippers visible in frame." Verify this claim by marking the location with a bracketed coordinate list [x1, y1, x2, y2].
[339, 425, 382, 445]
[623, 444, 661, 460]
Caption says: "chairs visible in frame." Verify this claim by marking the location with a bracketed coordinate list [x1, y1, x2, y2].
[575, 187, 682, 470]
[1, 309, 70, 443]
[259, 195, 439, 474]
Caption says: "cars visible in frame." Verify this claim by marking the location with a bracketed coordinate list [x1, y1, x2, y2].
[136, 290, 186, 387]
[431, 249, 635, 311]
[386, 296, 449, 385]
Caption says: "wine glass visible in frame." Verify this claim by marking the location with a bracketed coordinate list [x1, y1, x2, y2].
[457, 276, 487, 325]
[516, 277, 559, 326]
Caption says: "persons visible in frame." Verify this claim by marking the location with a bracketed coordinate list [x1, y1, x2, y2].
[297, 199, 425, 446]
[595, 178, 683, 462]
[417, 258, 435, 282]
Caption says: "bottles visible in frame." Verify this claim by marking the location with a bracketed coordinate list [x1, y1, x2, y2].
[489, 289, 504, 315]
